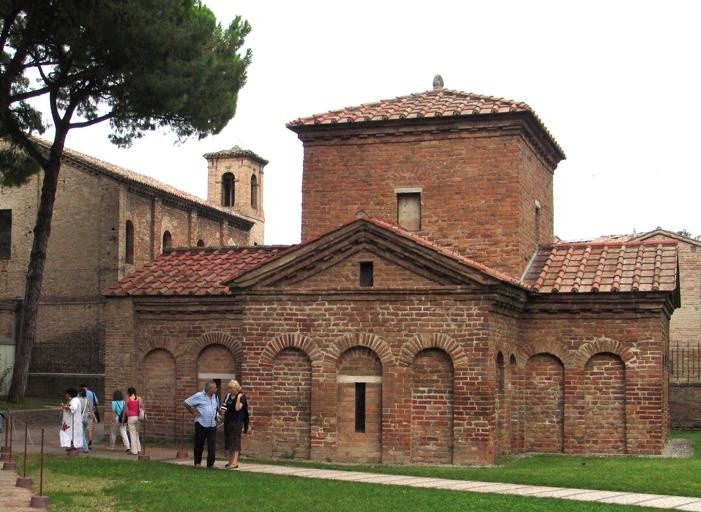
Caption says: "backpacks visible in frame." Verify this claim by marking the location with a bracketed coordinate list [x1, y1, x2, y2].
[115, 400, 128, 424]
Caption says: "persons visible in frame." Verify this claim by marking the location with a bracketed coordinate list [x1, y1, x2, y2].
[183, 381, 221, 471]
[54, 382, 99, 457]
[121, 386, 149, 456]
[104, 390, 131, 454]
[219, 379, 250, 471]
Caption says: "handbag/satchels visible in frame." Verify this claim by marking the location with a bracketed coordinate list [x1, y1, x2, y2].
[92, 408, 101, 424]
[139, 408, 145, 421]
[217, 404, 228, 424]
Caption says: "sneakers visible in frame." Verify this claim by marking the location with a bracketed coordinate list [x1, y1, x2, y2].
[194, 463, 239, 469]
